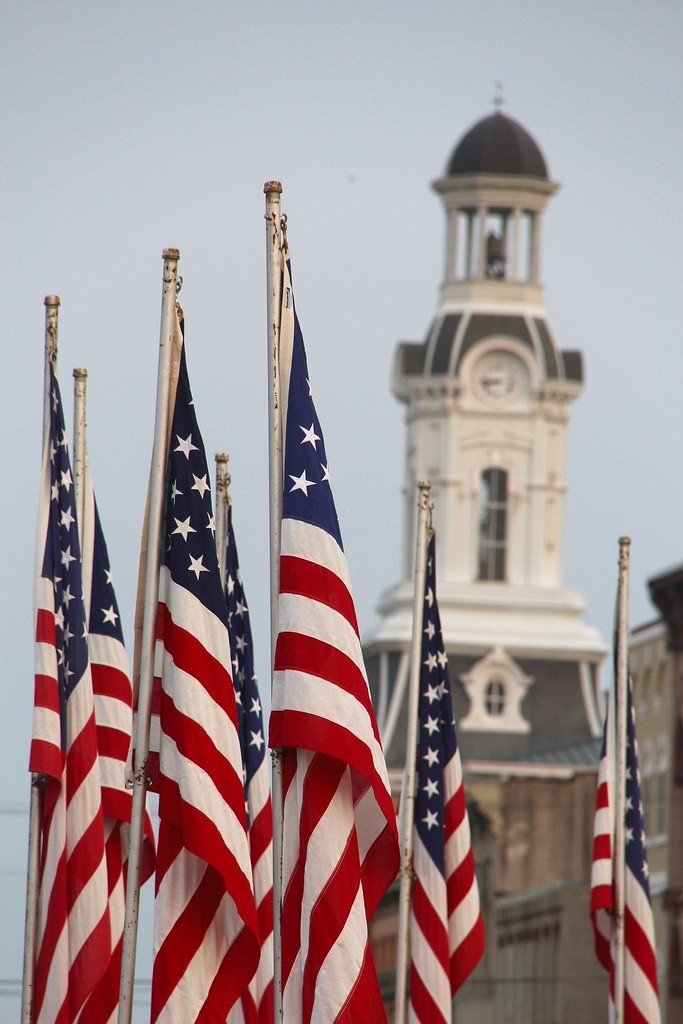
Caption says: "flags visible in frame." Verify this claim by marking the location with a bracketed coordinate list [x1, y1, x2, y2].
[268, 237, 402, 1024]
[134, 305, 277, 1024]
[406, 531, 487, 1024]
[29, 362, 157, 1024]
[588, 622, 663, 1024]
